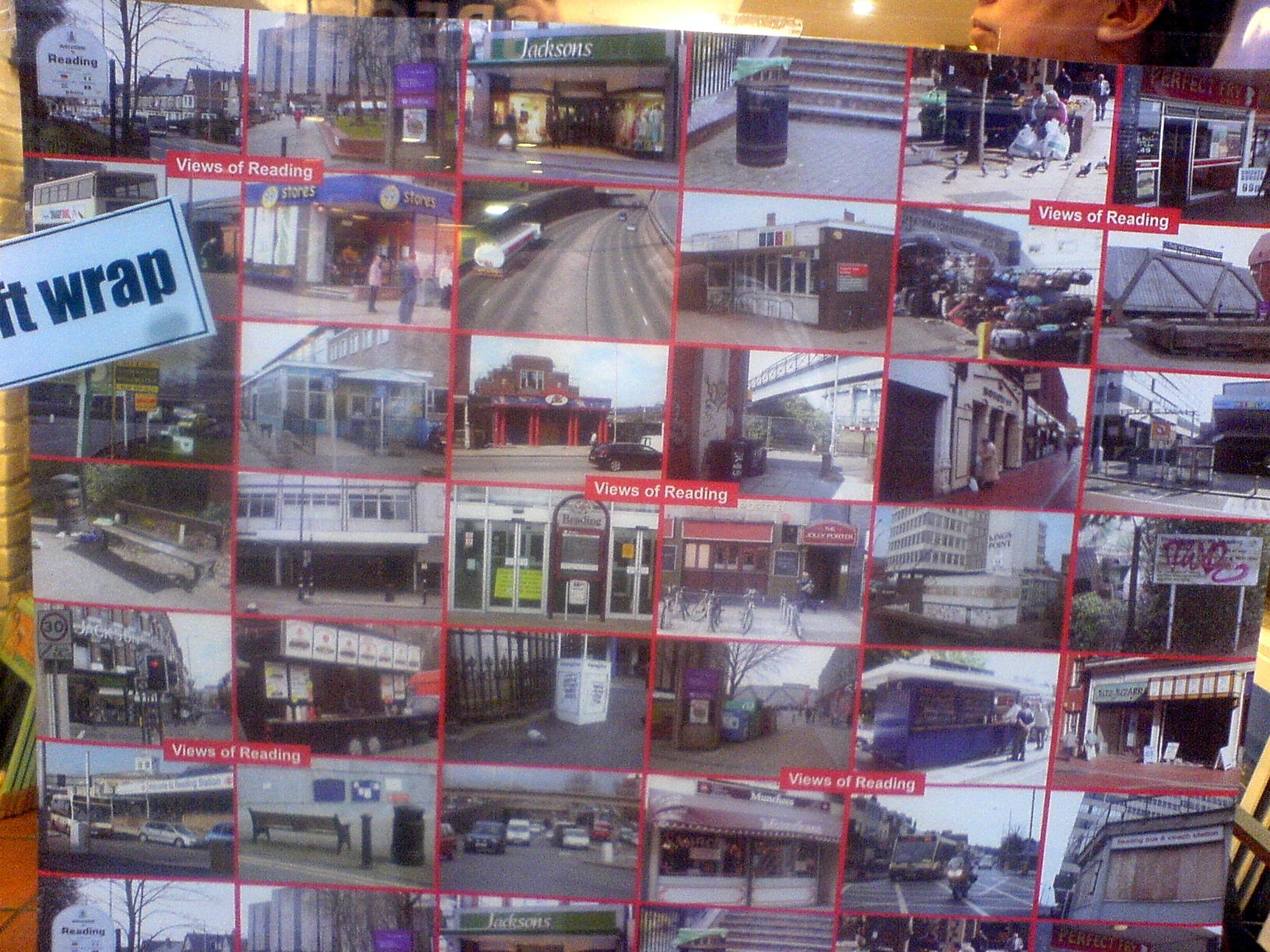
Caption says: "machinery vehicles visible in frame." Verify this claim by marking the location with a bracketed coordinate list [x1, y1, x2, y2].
[470, 219, 546, 280]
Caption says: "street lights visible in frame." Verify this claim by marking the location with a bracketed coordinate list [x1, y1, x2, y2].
[145, 652, 169, 694]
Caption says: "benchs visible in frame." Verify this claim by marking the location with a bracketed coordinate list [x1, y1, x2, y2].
[249, 808, 352, 857]
[97, 500, 225, 591]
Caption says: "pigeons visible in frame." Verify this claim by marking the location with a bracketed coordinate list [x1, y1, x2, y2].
[56, 529, 67, 540]
[527, 727, 549, 745]
[911, 142, 1109, 183]
[31, 538, 43, 548]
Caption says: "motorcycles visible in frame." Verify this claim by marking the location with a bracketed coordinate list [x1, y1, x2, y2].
[946, 865, 973, 901]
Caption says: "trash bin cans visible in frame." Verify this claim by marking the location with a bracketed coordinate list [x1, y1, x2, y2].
[735, 436, 767, 477]
[725, 700, 763, 739]
[709, 439, 744, 483]
[390, 806, 424, 866]
[388, 434, 408, 455]
[70, 822, 87, 851]
[918, 91, 950, 140]
[386, 584, 396, 602]
[1129, 456, 1139, 480]
[50, 473, 85, 536]
[720, 708, 752, 741]
[408, 667, 442, 715]
[206, 822, 234, 873]
[761, 706, 777, 734]
[736, 82, 790, 167]
[568, 579, 588, 606]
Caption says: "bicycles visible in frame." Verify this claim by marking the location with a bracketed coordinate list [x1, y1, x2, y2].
[659, 584, 725, 633]
[777, 589, 805, 639]
[738, 587, 767, 635]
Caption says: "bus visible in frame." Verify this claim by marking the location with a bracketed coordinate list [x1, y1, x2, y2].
[47, 791, 116, 839]
[887, 832, 961, 881]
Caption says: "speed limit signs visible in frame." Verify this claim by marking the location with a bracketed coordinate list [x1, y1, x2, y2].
[40, 612, 69, 641]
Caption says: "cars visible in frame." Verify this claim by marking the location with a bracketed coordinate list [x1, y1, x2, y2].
[589, 442, 662, 472]
[90, 114, 179, 139]
[137, 819, 201, 849]
[974, 855, 998, 870]
[205, 819, 234, 854]
[463, 818, 507, 855]
[504, 818, 532, 847]
[439, 822, 459, 863]
[527, 816, 639, 851]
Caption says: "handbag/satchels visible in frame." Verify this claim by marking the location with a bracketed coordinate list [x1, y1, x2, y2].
[1044, 127, 1071, 159]
[1006, 124, 1038, 160]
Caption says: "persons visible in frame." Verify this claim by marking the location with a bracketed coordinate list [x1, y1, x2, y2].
[1024, 81, 1072, 171]
[507, 105, 562, 153]
[624, 102, 664, 150]
[199, 236, 220, 273]
[398, 253, 419, 325]
[979, 439, 1000, 485]
[839, 917, 1026, 952]
[992, 68, 1021, 93]
[995, 697, 1049, 761]
[796, 570, 820, 613]
[289, 108, 305, 128]
[1089, 73, 1111, 121]
[1053, 67, 1073, 99]
[970, 0, 1167, 65]
[954, 842, 975, 877]
[367, 254, 386, 313]
